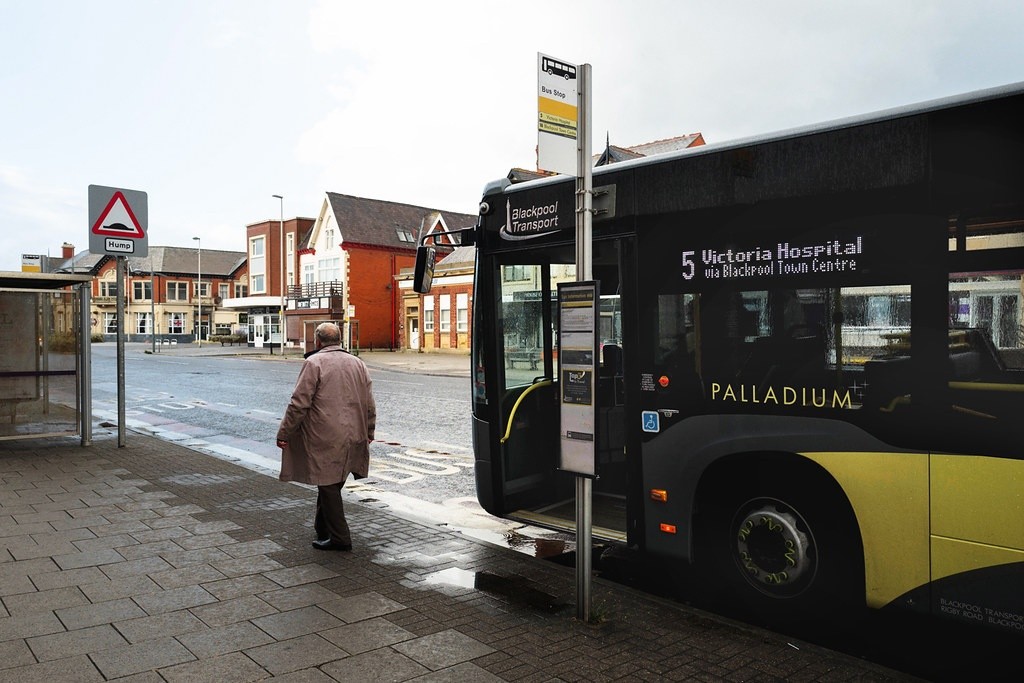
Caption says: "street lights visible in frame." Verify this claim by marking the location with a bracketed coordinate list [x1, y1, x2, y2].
[192, 237, 202, 347]
[272, 194, 285, 355]
[123, 255, 155, 354]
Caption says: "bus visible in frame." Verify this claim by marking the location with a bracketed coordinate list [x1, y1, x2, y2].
[410, 79, 1024, 660]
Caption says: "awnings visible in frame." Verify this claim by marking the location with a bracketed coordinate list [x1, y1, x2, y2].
[223, 297, 287, 308]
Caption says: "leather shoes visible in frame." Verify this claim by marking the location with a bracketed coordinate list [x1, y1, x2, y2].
[312, 539, 352, 551]
[317, 535, 329, 541]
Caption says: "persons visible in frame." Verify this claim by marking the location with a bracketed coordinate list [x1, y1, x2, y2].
[276, 323, 376, 551]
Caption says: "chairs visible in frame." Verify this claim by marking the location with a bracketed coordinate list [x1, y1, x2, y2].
[774, 320, 831, 396]
[860, 327, 987, 416]
